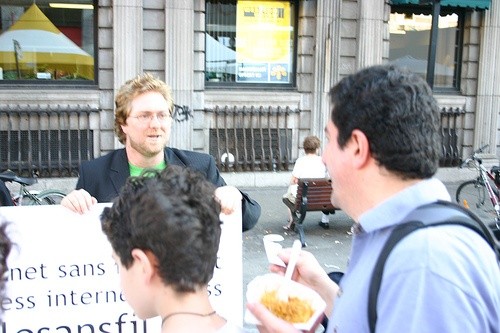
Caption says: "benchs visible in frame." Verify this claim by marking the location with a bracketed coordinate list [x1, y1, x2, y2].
[283, 178, 340, 246]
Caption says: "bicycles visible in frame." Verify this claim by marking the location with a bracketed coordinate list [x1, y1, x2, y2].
[0, 169, 67, 206]
[455, 144, 500, 242]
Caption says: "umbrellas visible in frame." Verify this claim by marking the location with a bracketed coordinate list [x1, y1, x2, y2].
[207, 33, 266, 73]
[0, 4, 93, 78]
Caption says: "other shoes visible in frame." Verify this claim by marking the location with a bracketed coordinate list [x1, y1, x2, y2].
[319, 220, 329, 229]
[282, 224, 291, 230]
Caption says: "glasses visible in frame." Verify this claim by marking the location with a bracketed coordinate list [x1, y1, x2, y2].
[127, 110, 171, 124]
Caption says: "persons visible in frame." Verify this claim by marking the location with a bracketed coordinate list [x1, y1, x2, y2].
[61, 76, 261, 232]
[284, 136, 330, 228]
[101, 166, 234, 333]
[247, 65, 500, 333]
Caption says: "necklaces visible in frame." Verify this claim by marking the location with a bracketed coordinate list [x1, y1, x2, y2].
[163, 310, 216, 322]
